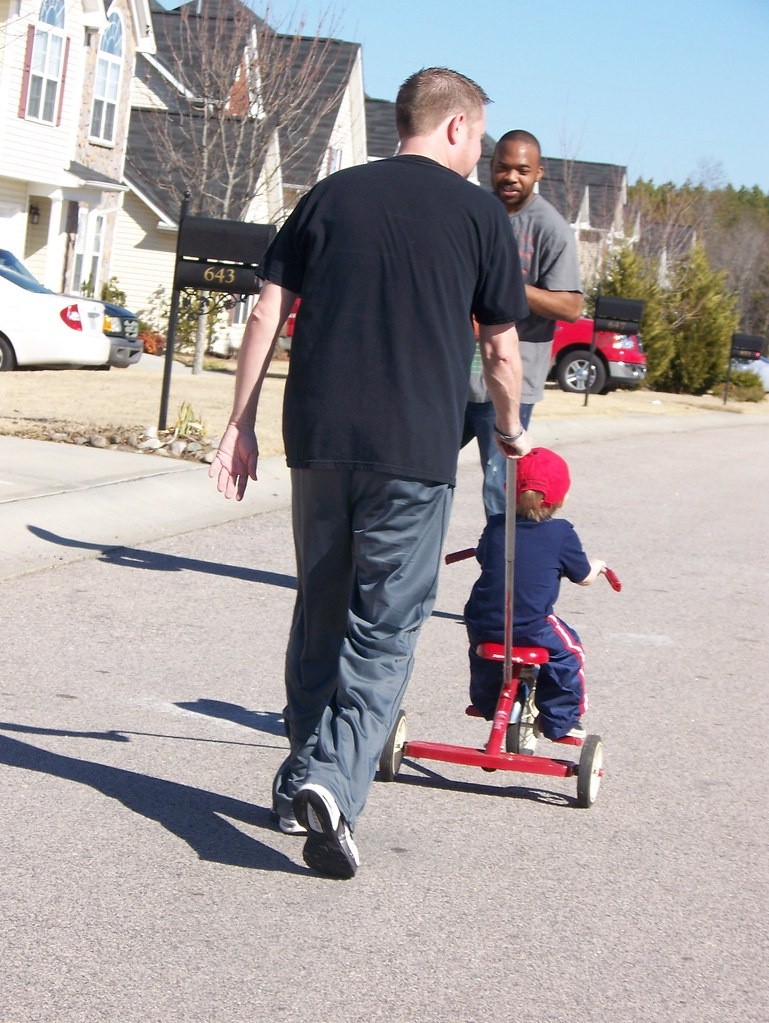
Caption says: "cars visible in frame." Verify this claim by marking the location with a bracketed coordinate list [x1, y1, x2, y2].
[280, 290, 649, 392]
[0, 249, 142, 371]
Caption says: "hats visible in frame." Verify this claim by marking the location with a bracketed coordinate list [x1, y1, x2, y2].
[504, 447, 570, 507]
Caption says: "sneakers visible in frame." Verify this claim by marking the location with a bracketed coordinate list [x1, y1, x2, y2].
[278, 816, 308, 836]
[292, 783, 360, 879]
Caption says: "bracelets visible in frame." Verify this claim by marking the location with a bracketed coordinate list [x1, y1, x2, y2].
[492, 422, 523, 442]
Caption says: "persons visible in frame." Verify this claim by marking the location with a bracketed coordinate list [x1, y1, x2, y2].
[462, 448, 607, 738]
[209, 68, 534, 880]
[457, 129, 582, 522]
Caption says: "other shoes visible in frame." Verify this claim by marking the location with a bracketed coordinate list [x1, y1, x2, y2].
[565, 721, 587, 738]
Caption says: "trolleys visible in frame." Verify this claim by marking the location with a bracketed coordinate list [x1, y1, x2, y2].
[376, 438, 606, 808]
